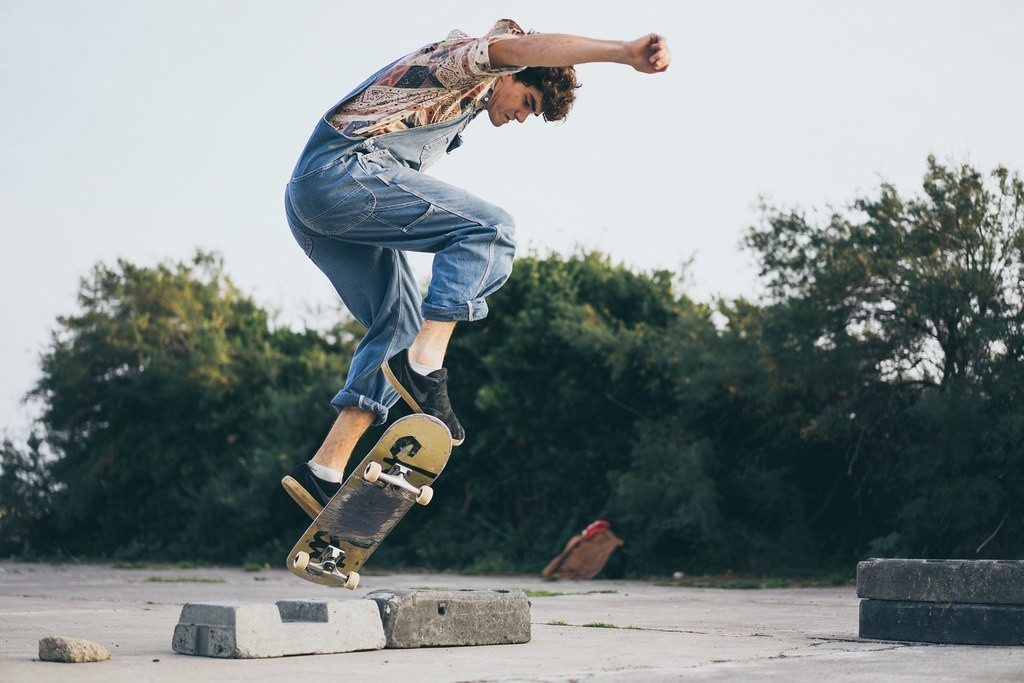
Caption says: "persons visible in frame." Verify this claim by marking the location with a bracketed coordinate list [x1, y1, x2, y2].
[280, 15, 672, 519]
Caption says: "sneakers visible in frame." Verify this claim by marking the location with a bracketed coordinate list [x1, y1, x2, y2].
[281, 462, 341, 521]
[380, 350, 467, 447]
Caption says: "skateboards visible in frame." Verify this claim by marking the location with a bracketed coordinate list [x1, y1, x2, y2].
[285, 412, 454, 591]
